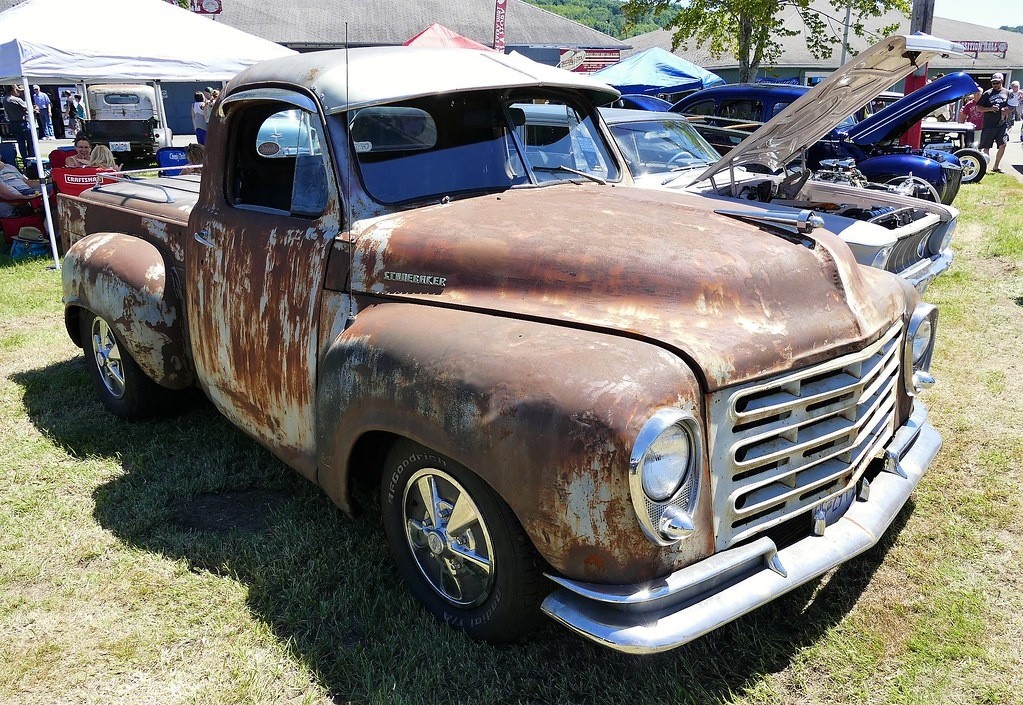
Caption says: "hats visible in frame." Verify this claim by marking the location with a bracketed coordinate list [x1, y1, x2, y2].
[11, 226, 49, 244]
[990, 72, 1003, 82]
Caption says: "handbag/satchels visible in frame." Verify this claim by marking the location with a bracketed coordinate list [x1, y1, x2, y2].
[11, 240, 48, 258]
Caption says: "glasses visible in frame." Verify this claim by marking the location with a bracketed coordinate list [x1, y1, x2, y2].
[991, 81, 1001, 85]
[76, 145, 91, 150]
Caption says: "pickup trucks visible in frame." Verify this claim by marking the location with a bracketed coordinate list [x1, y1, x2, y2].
[60, 20, 944, 655]
[80, 83, 174, 165]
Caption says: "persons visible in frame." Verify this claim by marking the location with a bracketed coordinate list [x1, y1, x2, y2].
[932, 73, 955, 122]
[1001, 81, 1023, 121]
[0, 83, 222, 221]
[976, 73, 1015, 175]
[960, 87, 986, 148]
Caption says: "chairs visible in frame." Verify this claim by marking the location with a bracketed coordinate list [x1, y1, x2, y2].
[0, 143, 190, 245]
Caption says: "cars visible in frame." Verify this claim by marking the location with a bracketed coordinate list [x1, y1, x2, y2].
[861, 91, 988, 185]
[504, 30, 975, 298]
[615, 71, 978, 209]
